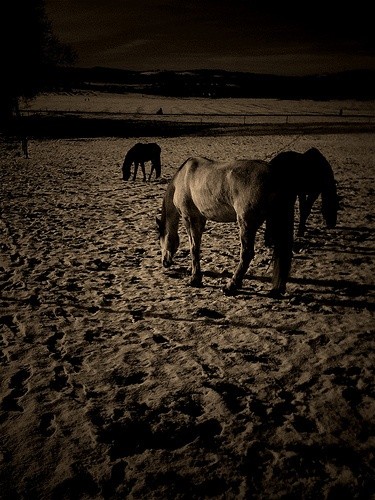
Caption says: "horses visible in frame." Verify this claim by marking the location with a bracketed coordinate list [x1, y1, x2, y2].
[119, 141, 162, 183]
[153, 156, 296, 299]
[264, 147, 339, 247]
